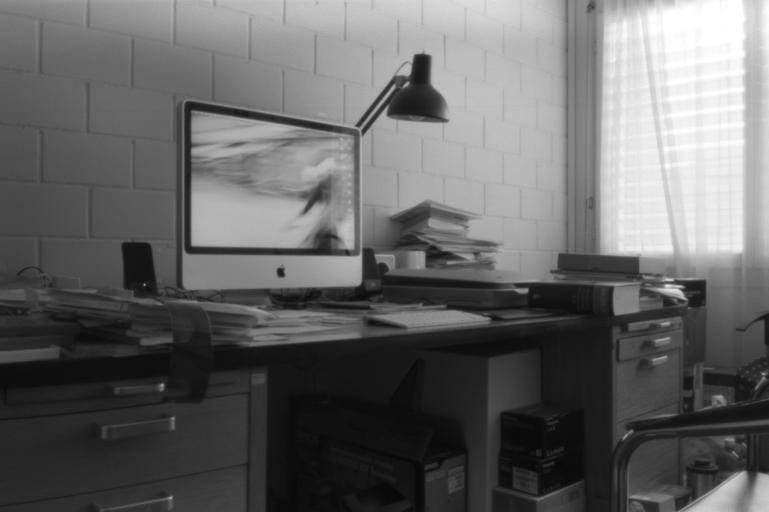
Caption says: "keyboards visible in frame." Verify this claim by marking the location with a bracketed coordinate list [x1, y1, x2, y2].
[364, 309, 492, 331]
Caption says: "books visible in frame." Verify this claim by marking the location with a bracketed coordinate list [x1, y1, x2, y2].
[388, 197, 505, 270]
[1, 280, 491, 365]
[520, 251, 701, 318]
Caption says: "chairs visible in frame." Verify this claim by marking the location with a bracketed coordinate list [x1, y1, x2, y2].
[609, 370, 768, 512]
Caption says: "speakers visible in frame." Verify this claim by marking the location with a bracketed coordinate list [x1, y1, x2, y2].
[121, 241, 158, 293]
[362, 247, 381, 294]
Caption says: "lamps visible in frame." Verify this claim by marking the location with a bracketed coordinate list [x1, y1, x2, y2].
[356, 51, 450, 136]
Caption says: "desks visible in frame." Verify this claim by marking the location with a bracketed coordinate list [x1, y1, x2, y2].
[0, 303, 688, 512]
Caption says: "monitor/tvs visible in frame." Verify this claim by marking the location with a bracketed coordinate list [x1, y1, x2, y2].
[175, 99, 363, 289]
